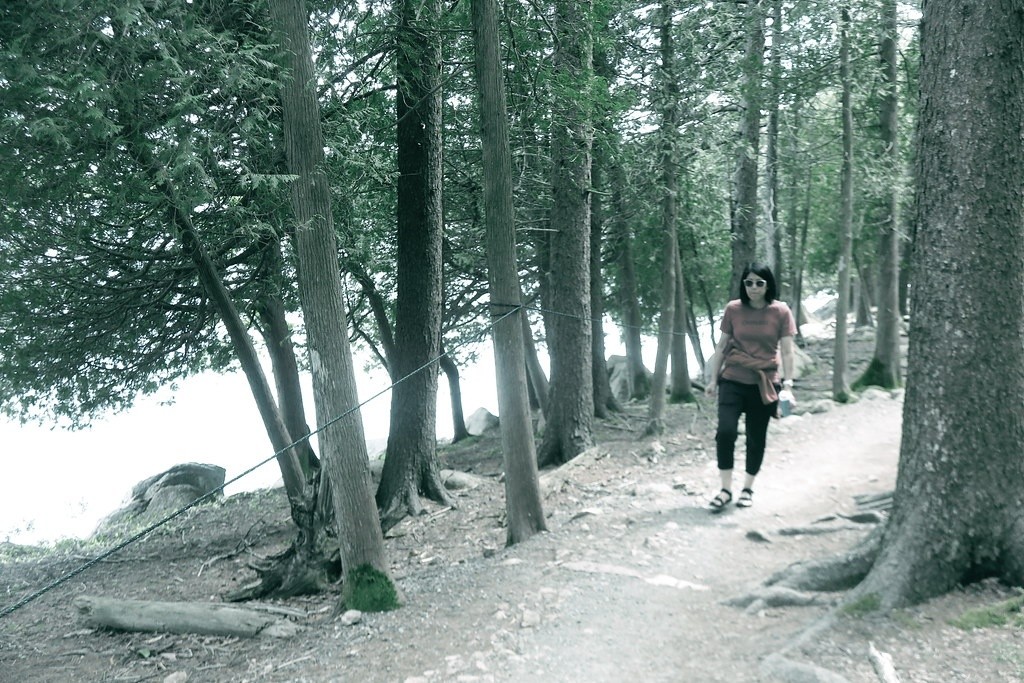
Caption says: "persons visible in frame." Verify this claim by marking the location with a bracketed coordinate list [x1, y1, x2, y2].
[704, 262, 799, 509]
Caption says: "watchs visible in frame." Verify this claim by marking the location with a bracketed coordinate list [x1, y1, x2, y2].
[783, 380, 793, 386]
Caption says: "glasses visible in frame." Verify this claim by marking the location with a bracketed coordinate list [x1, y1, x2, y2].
[743, 279, 767, 287]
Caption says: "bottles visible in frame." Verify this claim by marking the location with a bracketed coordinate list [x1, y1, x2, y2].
[778, 390, 790, 417]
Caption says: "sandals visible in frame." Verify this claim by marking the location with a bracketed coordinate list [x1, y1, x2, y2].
[737, 488, 753, 507]
[710, 489, 732, 507]
